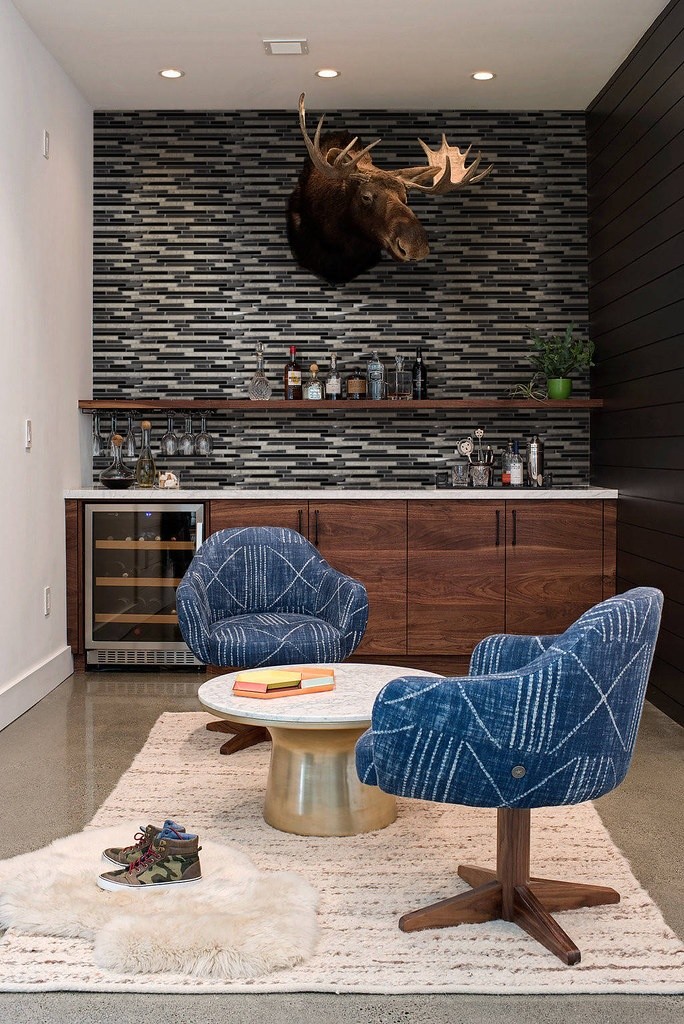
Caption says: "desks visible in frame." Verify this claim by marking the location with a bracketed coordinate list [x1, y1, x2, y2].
[198, 662, 447, 837]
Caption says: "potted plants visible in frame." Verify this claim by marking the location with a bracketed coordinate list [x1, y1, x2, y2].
[527, 322, 595, 401]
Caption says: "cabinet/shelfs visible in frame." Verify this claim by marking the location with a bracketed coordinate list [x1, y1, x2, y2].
[80, 498, 203, 662]
[206, 499, 407, 657]
[408, 497, 619, 655]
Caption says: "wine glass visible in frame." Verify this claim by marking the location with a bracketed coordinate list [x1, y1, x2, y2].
[93, 412, 214, 456]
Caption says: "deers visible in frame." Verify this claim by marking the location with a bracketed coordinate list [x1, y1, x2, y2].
[286, 90, 495, 285]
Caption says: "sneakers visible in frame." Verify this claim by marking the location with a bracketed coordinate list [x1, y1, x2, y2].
[96, 829, 202, 893]
[103, 820, 185, 869]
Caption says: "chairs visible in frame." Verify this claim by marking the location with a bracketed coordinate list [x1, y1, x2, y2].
[175, 525, 368, 756]
[354, 587, 664, 964]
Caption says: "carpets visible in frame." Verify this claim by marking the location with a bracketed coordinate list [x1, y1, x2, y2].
[0, 711, 684, 999]
[0, 822, 319, 978]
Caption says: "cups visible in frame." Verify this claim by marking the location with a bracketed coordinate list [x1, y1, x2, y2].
[158, 470, 180, 490]
[469, 462, 494, 489]
[451, 465, 469, 488]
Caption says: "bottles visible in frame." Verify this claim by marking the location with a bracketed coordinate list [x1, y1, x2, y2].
[102, 528, 187, 642]
[526, 435, 544, 487]
[284, 345, 303, 400]
[303, 364, 325, 400]
[387, 355, 413, 400]
[345, 366, 366, 400]
[501, 439, 514, 487]
[413, 347, 427, 400]
[99, 435, 137, 489]
[248, 343, 272, 401]
[325, 354, 343, 400]
[134, 420, 156, 487]
[510, 441, 523, 487]
[367, 351, 387, 400]
